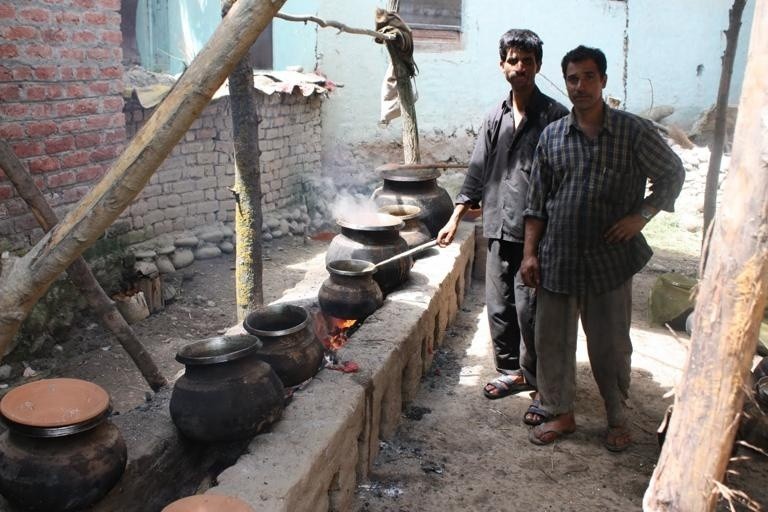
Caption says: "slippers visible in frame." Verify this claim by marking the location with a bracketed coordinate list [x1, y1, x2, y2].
[483, 375, 531, 398]
[524, 399, 576, 445]
[606, 426, 629, 451]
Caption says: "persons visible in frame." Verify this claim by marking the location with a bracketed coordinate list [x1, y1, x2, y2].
[438, 28, 570, 427]
[520, 44, 685, 452]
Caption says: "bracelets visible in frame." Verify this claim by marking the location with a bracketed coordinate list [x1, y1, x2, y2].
[633, 208, 653, 221]
